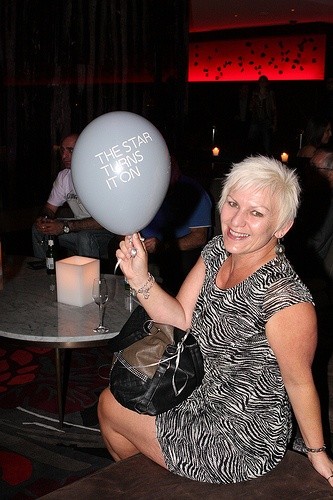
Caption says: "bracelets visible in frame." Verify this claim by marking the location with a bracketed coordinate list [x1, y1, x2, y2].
[130, 272, 151, 296]
[303, 444, 327, 453]
[140, 276, 155, 299]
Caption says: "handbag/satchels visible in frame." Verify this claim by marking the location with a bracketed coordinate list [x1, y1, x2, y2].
[98, 320, 206, 416]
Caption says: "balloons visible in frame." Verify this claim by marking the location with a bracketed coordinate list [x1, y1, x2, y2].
[71, 111, 171, 242]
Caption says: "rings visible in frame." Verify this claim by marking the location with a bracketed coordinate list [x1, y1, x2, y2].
[327, 475, 333, 481]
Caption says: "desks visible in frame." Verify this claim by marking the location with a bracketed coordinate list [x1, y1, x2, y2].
[0, 254, 140, 430]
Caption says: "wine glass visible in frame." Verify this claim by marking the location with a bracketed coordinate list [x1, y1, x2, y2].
[92, 278, 109, 333]
[38, 216, 48, 246]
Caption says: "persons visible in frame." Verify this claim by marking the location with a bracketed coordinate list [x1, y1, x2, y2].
[98, 155, 333, 488]
[32, 78, 333, 289]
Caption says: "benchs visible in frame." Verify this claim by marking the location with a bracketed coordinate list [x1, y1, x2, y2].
[38, 450, 333, 500]
[19, 206, 113, 260]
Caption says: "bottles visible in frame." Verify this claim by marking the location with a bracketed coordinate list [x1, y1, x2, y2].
[46, 234, 56, 275]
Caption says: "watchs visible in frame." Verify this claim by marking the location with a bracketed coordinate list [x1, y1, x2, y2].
[62, 220, 70, 235]
